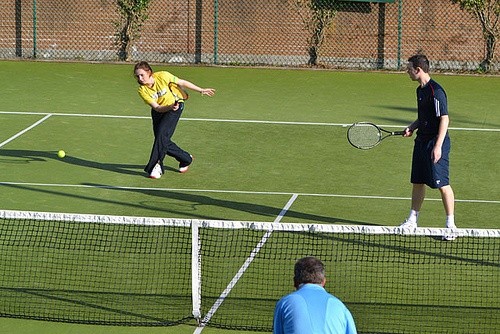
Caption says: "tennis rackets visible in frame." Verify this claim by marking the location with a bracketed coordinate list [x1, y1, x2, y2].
[347, 122, 413, 150]
[168, 82, 188, 111]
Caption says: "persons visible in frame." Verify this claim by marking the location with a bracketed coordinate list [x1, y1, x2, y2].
[273, 256, 357, 334]
[133, 61, 216, 179]
[395, 54, 459, 241]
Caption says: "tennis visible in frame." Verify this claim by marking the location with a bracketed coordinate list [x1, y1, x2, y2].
[58, 150, 65, 158]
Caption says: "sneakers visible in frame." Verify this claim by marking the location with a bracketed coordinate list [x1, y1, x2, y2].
[150, 164, 162, 179]
[442, 225, 458, 240]
[400, 219, 417, 227]
[179, 155, 193, 173]
